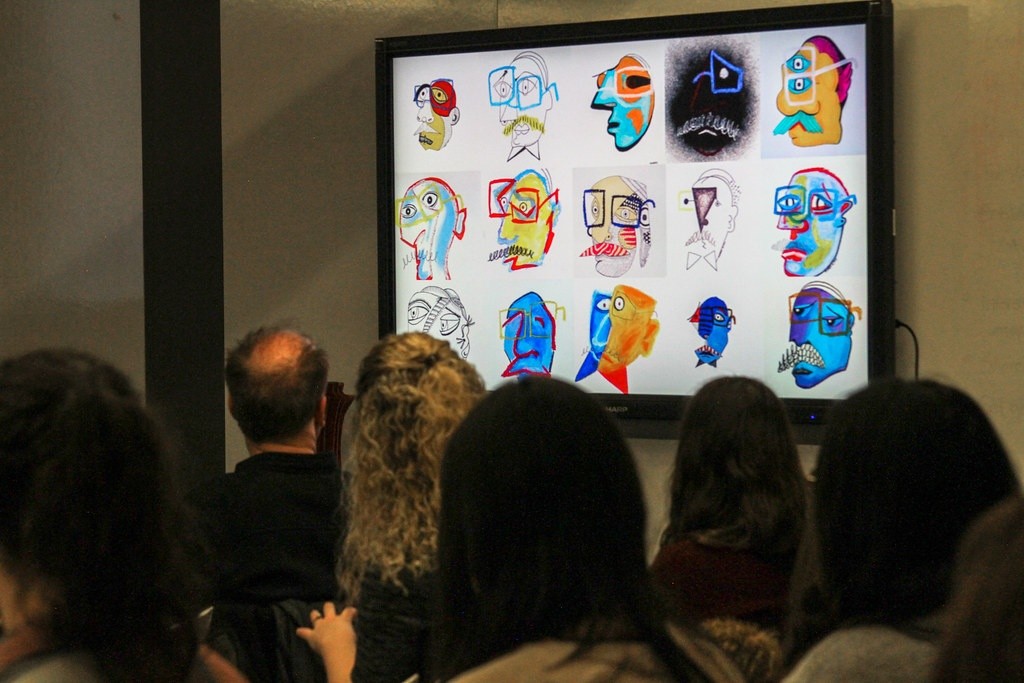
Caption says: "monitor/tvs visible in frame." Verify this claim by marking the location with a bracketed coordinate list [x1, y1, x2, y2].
[377, 0, 895, 424]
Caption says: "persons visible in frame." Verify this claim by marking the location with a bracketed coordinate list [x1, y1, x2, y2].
[297, 375, 745, 683]
[650, 375, 814, 636]
[186, 327, 486, 683]
[781, 378, 1024, 683]
[0, 347, 248, 683]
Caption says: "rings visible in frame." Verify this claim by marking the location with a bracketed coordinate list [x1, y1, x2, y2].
[310, 616, 322, 624]
